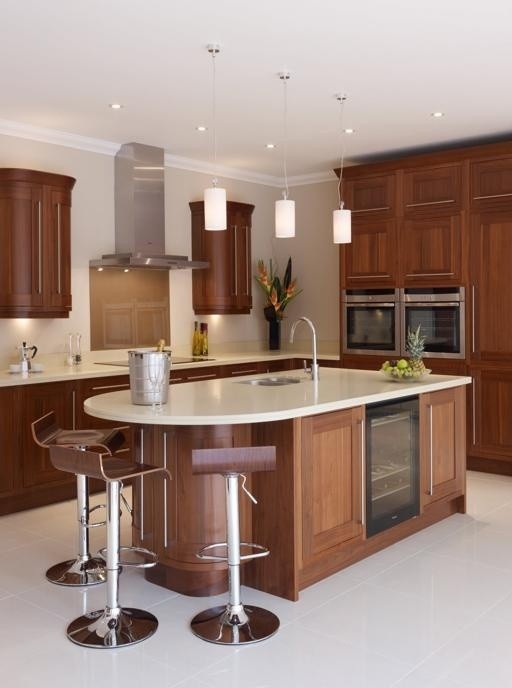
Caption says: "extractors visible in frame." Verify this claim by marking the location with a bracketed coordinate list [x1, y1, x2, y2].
[88, 142, 212, 272]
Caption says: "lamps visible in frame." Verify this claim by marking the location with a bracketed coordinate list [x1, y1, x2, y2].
[203, 44, 229, 232]
[274, 72, 296, 238]
[333, 93, 352, 245]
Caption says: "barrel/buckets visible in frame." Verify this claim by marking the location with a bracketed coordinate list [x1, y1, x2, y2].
[128, 350, 170, 406]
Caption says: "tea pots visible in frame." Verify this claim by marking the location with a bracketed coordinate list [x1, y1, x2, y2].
[16, 340, 39, 372]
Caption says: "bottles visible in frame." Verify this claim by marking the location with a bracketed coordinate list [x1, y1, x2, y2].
[60, 331, 87, 367]
[191, 320, 209, 356]
[152, 337, 168, 353]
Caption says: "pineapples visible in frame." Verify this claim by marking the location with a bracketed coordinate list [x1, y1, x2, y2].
[406, 322, 425, 374]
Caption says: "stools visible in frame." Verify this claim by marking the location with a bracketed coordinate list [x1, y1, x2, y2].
[47, 444, 172, 650]
[30, 411, 125, 586]
[190, 444, 277, 646]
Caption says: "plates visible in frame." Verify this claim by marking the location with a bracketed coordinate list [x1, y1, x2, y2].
[377, 368, 433, 383]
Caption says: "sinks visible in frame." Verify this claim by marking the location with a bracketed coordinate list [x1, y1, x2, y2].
[237, 376, 299, 387]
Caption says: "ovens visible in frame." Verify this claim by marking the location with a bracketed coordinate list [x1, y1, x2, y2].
[338, 284, 467, 359]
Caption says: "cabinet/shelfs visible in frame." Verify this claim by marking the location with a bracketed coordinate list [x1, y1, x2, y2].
[132, 426, 236, 572]
[0, 379, 76, 516]
[1, 168, 76, 319]
[418, 383, 466, 530]
[296, 404, 367, 593]
[190, 200, 253, 315]
[82, 374, 140, 492]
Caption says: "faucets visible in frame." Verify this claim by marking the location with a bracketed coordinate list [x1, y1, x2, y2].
[289, 317, 320, 382]
[303, 385, 318, 407]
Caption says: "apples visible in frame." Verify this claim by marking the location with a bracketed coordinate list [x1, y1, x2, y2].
[382, 359, 411, 374]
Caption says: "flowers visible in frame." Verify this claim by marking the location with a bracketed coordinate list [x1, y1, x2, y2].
[253, 256, 303, 324]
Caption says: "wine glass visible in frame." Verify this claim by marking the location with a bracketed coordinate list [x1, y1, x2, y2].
[147, 354, 172, 416]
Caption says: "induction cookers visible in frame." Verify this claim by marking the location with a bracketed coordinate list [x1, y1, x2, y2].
[92, 355, 216, 368]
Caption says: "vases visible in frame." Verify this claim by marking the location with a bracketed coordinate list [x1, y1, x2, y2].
[268, 319, 281, 349]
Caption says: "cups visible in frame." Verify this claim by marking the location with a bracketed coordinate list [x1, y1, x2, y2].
[9, 365, 21, 374]
